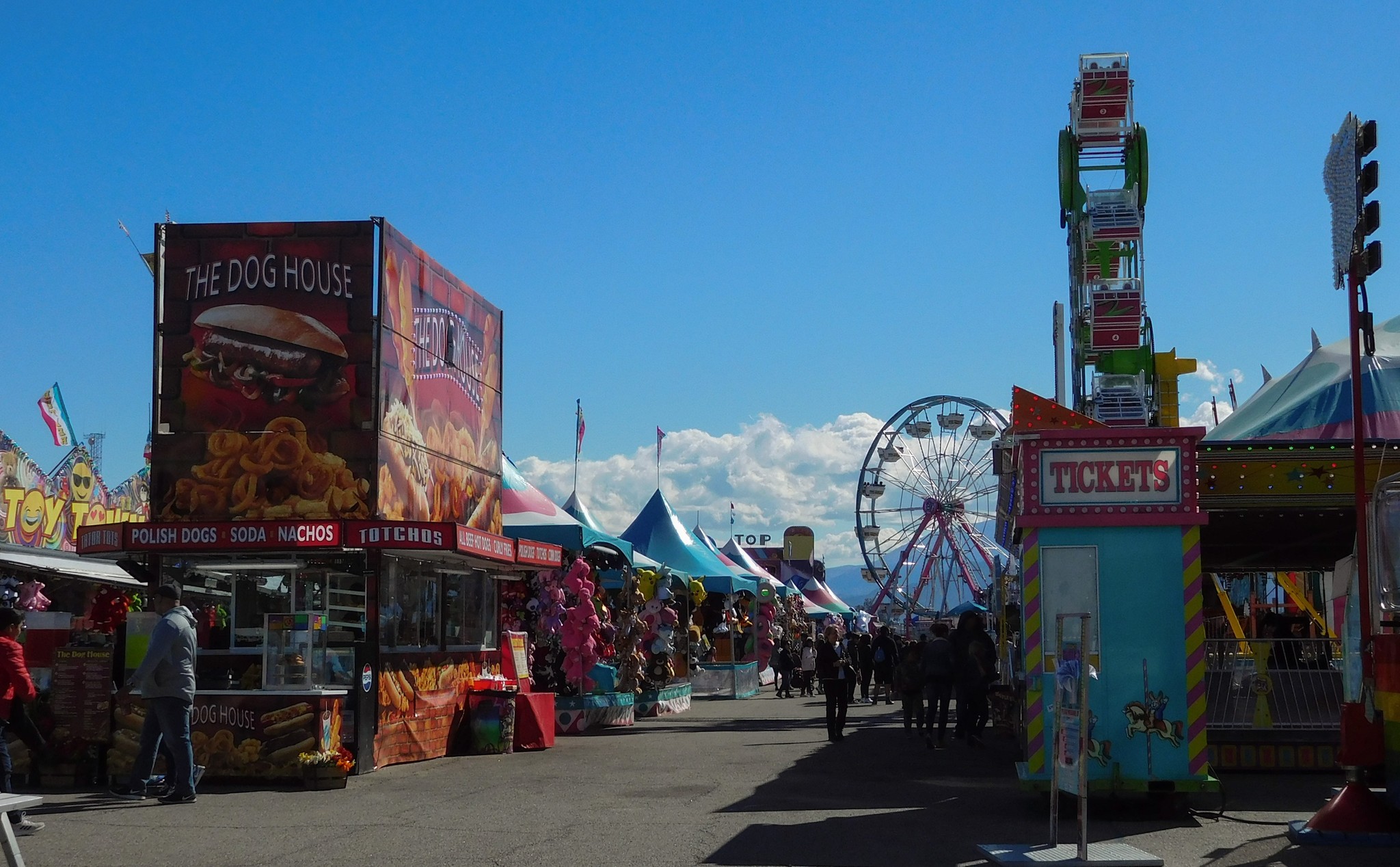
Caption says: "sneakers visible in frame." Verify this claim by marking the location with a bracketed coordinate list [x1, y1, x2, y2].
[192, 764, 206, 788]
[848, 699, 859, 704]
[860, 697, 874, 703]
[9, 811, 46, 837]
[157, 791, 197, 804]
[151, 785, 176, 799]
[108, 785, 147, 800]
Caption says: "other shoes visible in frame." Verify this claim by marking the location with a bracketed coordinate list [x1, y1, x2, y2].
[926, 739, 947, 751]
[800, 694, 808, 698]
[789, 687, 794, 690]
[971, 734, 984, 745]
[829, 731, 844, 744]
[906, 734, 914, 739]
[919, 730, 923, 736]
[810, 694, 816, 697]
[775, 686, 779, 691]
[815, 685, 825, 695]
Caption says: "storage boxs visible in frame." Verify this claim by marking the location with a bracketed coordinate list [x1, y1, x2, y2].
[474, 680, 517, 690]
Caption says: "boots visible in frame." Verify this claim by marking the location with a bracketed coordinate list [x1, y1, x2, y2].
[776, 689, 782, 698]
[785, 691, 794, 698]
[885, 696, 895, 705]
[872, 697, 878, 705]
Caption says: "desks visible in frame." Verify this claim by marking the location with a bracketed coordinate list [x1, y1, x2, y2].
[0, 793, 43, 867]
[515, 692, 555, 750]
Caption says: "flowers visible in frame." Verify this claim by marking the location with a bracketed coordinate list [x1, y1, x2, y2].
[298, 746, 356, 772]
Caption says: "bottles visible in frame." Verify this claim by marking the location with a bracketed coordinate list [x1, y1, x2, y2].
[491, 676, 498, 690]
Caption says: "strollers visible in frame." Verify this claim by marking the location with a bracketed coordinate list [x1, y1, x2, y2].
[790, 653, 815, 690]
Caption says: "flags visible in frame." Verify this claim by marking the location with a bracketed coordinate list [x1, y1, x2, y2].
[730, 502, 735, 523]
[143, 433, 152, 460]
[658, 428, 666, 466]
[578, 407, 585, 452]
[38, 385, 76, 446]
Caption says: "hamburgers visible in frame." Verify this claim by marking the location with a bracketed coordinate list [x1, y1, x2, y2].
[183, 305, 349, 401]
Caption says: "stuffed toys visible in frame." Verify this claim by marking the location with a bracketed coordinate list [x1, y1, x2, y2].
[502, 558, 775, 697]
[0, 568, 226, 649]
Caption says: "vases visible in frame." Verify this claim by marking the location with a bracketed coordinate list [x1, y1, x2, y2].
[303, 767, 348, 791]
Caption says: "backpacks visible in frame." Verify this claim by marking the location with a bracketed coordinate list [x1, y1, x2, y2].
[873, 636, 892, 666]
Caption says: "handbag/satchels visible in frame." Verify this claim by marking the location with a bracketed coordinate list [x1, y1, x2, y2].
[782, 652, 796, 669]
[845, 665, 856, 682]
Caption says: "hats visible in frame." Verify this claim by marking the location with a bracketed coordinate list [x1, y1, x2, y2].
[155, 584, 182, 600]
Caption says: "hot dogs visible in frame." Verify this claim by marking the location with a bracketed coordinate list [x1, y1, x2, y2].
[262, 728, 315, 763]
[456, 437, 503, 529]
[114, 704, 146, 734]
[383, 662, 414, 710]
[261, 702, 314, 734]
[436, 657, 454, 688]
[382, 401, 436, 524]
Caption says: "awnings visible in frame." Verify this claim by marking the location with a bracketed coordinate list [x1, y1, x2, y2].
[0, 549, 233, 596]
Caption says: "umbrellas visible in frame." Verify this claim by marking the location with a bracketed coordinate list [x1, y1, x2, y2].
[945, 601, 988, 617]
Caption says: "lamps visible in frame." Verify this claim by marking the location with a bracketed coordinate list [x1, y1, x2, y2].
[191, 559, 301, 570]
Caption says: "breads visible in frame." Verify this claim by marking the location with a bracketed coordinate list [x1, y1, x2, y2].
[287, 653, 305, 666]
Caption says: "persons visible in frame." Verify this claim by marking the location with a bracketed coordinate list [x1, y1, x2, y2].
[0, 607, 45, 835]
[109, 584, 200, 804]
[768, 605, 1022, 751]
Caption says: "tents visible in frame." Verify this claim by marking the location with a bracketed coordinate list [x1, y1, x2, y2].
[502, 451, 633, 733]
[562, 489, 859, 717]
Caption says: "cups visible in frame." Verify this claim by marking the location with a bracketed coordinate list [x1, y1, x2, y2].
[322, 710, 331, 750]
